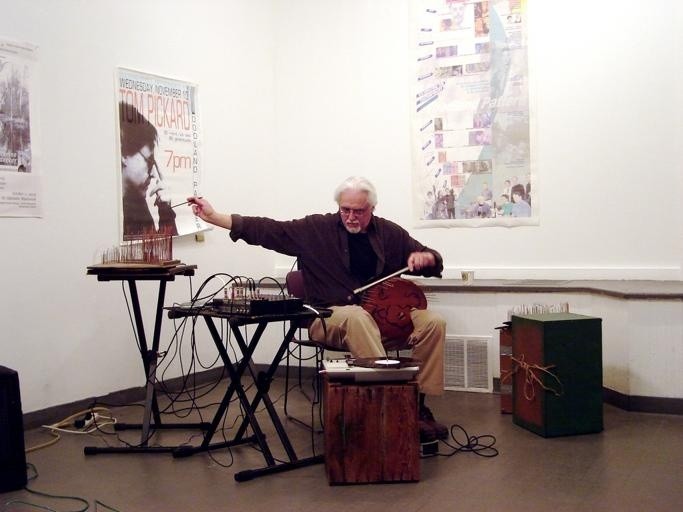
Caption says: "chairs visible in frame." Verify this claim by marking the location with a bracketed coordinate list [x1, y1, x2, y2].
[282, 270, 414, 435]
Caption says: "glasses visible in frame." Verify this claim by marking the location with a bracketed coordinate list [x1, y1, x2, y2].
[340, 208, 366, 215]
[138, 150, 154, 169]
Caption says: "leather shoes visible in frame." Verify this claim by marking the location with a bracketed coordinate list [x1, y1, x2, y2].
[419, 405, 449, 442]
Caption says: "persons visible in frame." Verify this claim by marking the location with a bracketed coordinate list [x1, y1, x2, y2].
[432, 111, 494, 173]
[120, 101, 177, 240]
[440, 0, 523, 37]
[463, 173, 532, 219]
[424, 180, 457, 219]
[186, 174, 451, 441]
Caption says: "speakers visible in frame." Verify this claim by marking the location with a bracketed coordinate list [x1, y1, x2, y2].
[0, 364, 29, 494]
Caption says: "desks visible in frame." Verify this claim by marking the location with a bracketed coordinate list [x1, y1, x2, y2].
[83, 262, 214, 457]
[322, 376, 423, 486]
[509, 313, 605, 439]
[168, 304, 335, 483]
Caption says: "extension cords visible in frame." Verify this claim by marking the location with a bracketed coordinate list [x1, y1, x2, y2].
[74, 412, 99, 431]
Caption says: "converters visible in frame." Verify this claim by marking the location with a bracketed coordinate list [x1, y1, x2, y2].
[421, 438, 440, 454]
[74, 418, 84, 427]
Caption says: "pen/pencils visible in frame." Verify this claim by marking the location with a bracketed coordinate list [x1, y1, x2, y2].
[168, 196, 203, 209]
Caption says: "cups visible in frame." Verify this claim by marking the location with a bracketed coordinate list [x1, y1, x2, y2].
[461, 270, 474, 285]
